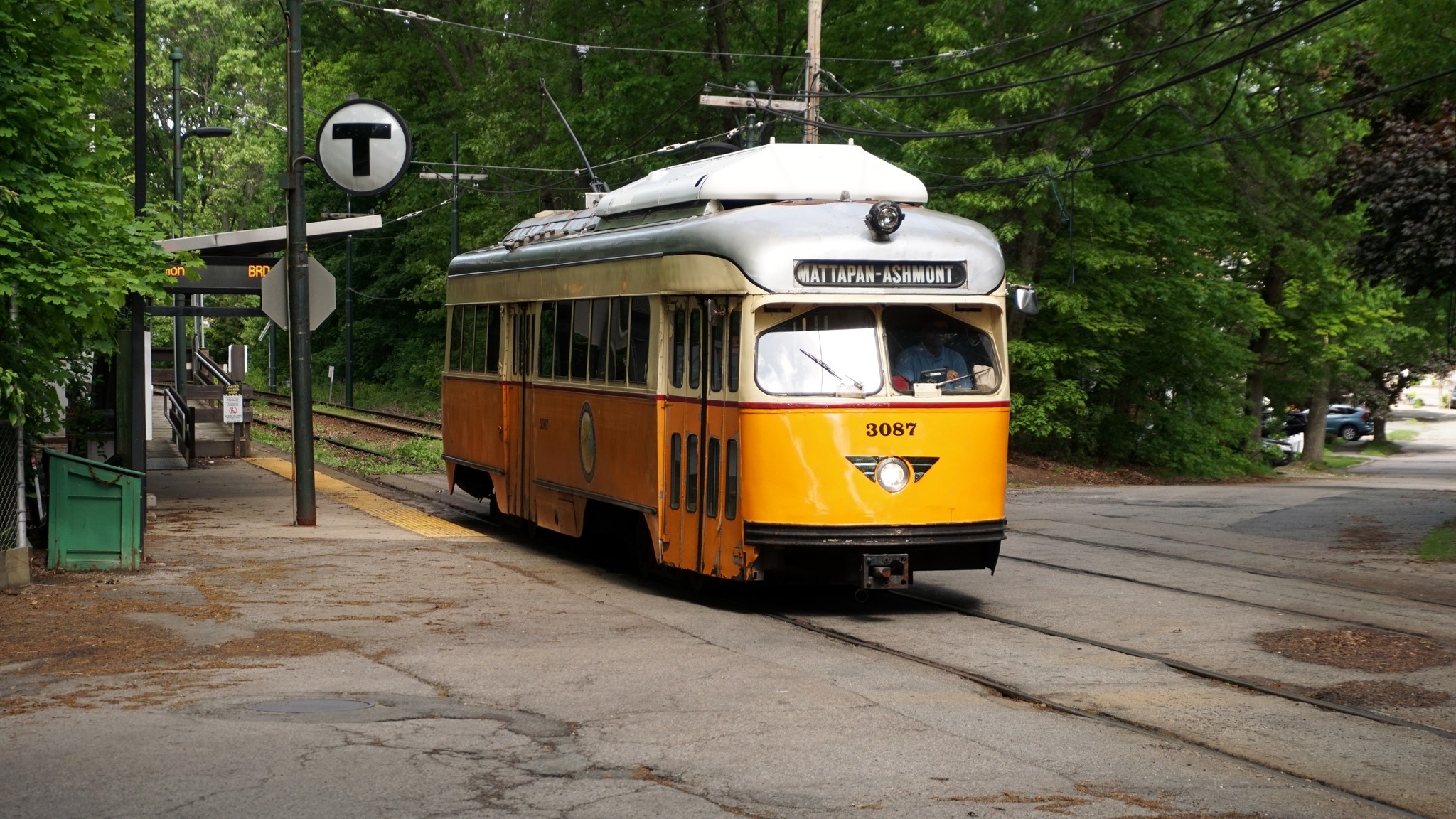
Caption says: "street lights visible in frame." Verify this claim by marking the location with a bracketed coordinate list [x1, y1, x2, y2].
[172, 127, 236, 396]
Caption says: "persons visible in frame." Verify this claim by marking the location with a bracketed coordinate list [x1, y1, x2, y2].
[897, 311, 972, 387]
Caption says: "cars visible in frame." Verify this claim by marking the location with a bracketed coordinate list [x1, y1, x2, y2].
[1301, 404, 1375, 441]
[1137, 409, 1309, 466]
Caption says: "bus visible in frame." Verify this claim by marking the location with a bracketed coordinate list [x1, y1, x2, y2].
[438, 77, 1039, 593]
